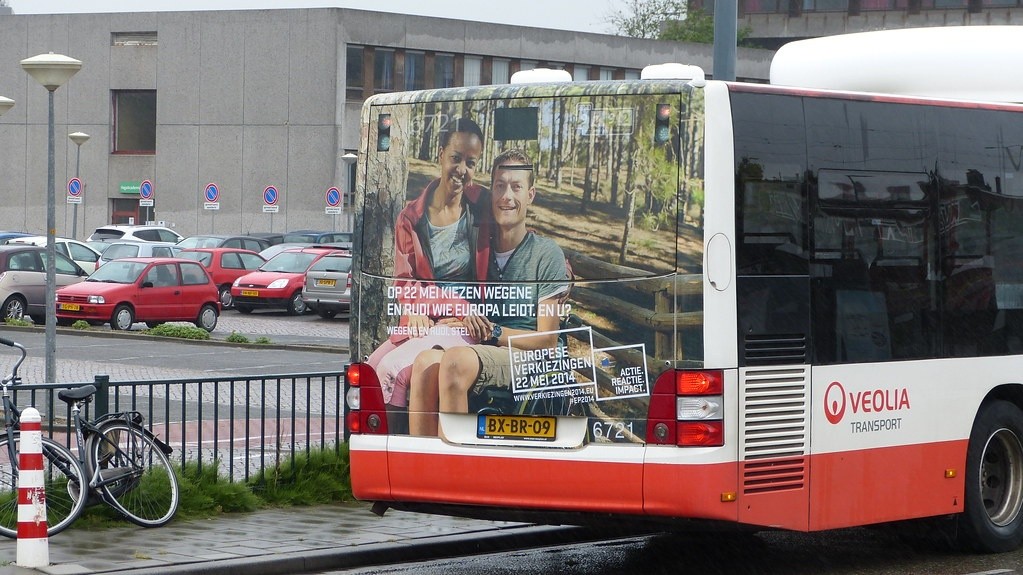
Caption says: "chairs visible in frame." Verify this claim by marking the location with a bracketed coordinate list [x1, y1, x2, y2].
[184, 275, 197, 281]
[20, 256, 32, 267]
[136, 270, 148, 281]
[206, 243, 214, 249]
[157, 268, 170, 282]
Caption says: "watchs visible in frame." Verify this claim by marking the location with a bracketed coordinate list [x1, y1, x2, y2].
[483, 324, 502, 346]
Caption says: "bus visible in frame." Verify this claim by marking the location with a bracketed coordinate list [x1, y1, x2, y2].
[343, 61, 1023, 557]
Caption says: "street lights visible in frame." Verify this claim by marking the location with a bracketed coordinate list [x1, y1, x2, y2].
[341, 152, 358, 232]
[18, 52, 84, 422]
[68, 131, 92, 239]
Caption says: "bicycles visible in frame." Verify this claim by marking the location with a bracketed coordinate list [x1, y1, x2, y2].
[0, 335, 180, 539]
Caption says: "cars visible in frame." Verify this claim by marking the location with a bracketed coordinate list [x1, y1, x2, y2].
[0, 224, 353, 275]
[230, 247, 335, 317]
[172, 246, 268, 310]
[300, 251, 352, 319]
[55, 257, 222, 334]
[0, 240, 90, 325]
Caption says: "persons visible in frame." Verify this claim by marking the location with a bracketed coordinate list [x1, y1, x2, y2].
[363, 118, 575, 438]
